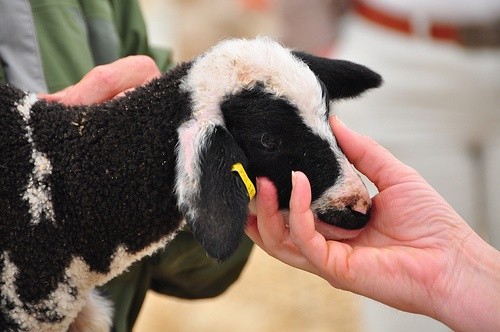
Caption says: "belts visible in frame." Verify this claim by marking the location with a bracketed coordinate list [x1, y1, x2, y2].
[349, 1, 499, 50]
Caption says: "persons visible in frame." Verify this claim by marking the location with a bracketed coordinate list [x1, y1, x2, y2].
[245, 111, 500, 331]
[0, 0, 257, 332]
[276, 0, 499, 332]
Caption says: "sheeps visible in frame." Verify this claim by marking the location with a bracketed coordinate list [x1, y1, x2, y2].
[1, 33, 386, 332]
